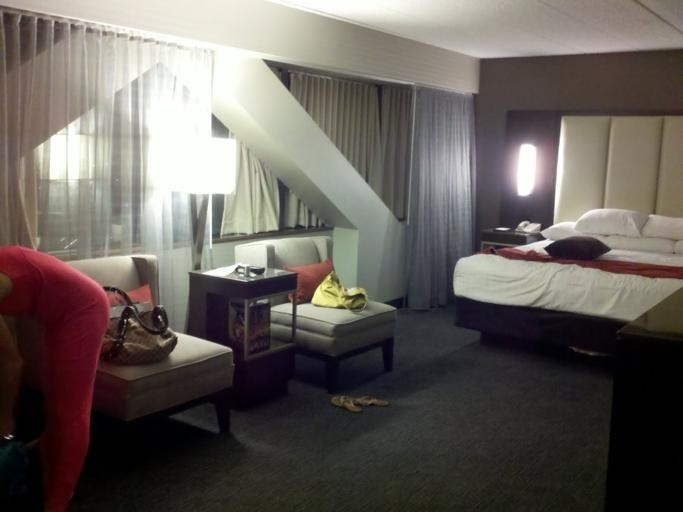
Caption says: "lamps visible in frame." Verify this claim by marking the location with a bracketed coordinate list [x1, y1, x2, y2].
[172, 135, 242, 267]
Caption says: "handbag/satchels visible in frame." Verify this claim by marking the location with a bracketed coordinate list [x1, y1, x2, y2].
[310, 271, 368, 310]
[101, 311, 177, 365]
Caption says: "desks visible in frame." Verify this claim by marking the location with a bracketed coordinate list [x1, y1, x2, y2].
[186, 265, 299, 412]
[599, 288, 682, 512]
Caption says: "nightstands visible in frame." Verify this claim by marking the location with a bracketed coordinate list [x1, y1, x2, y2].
[480, 225, 540, 252]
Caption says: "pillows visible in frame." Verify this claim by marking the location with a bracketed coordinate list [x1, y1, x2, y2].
[282, 258, 335, 306]
[539, 207, 683, 264]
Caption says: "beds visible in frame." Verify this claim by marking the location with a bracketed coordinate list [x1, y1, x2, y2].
[450, 110, 682, 361]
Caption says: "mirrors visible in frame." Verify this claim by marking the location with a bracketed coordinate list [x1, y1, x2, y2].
[104, 283, 153, 306]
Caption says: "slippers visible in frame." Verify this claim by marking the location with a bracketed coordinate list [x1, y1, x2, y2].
[331, 394, 389, 413]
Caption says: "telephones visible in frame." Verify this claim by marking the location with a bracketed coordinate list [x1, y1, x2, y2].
[514, 220, 542, 234]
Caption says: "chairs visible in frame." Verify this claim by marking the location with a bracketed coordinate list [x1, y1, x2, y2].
[61, 251, 235, 451]
[235, 233, 397, 394]
